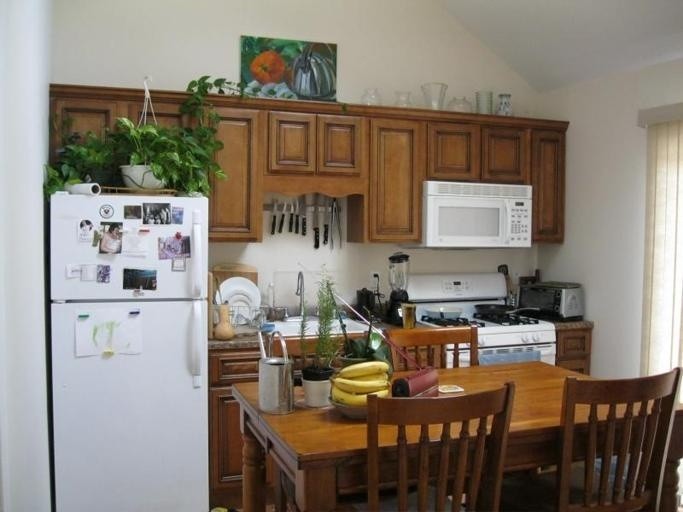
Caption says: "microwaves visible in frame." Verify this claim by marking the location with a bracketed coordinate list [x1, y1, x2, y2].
[421, 176, 533, 249]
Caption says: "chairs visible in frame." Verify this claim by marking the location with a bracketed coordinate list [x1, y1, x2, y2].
[361, 381, 518, 512]
[517, 365, 681, 512]
[388, 326, 482, 371]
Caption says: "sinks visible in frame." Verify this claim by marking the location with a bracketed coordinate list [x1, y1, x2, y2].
[263, 316, 379, 338]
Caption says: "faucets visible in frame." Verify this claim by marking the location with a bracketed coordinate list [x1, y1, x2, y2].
[295, 271, 305, 315]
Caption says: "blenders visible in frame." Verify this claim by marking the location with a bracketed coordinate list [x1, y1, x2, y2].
[388, 247, 414, 327]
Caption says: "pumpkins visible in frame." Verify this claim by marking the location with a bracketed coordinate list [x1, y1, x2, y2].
[250, 50, 285, 84]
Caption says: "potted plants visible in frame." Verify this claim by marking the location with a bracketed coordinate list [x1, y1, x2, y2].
[39, 74, 294, 196]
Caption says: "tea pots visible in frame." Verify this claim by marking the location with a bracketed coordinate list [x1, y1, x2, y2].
[284, 42, 336, 101]
[254, 326, 296, 415]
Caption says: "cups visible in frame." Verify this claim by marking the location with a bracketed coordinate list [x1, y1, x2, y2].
[360, 77, 514, 117]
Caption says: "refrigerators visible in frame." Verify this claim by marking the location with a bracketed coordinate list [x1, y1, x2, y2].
[47, 182, 213, 510]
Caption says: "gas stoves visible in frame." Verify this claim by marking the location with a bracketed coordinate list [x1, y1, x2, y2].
[415, 301, 558, 344]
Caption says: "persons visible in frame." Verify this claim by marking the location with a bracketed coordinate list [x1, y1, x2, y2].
[146, 206, 170, 224]
[79, 219, 93, 242]
[100, 223, 121, 253]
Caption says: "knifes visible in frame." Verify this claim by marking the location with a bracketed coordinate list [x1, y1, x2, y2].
[269, 194, 331, 248]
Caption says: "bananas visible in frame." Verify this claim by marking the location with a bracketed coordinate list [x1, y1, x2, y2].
[330, 360, 392, 406]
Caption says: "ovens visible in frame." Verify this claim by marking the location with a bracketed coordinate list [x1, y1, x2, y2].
[446, 341, 558, 368]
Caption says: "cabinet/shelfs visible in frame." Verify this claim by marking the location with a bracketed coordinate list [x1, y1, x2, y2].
[530, 118, 569, 245]
[557, 329, 592, 373]
[427, 110, 530, 185]
[49, 83, 265, 244]
[349, 105, 427, 243]
[266, 99, 368, 198]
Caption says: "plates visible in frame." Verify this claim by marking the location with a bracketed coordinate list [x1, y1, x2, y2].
[215, 276, 264, 326]
[325, 392, 370, 421]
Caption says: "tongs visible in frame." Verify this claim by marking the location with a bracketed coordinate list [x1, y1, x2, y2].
[329, 199, 343, 250]
